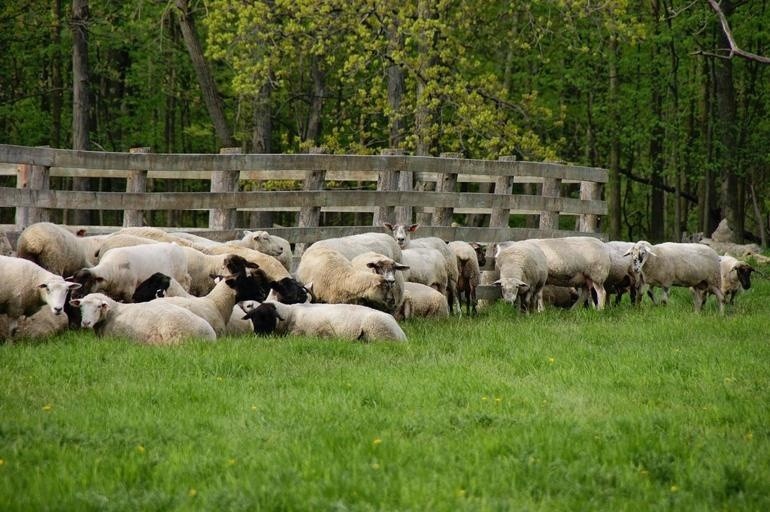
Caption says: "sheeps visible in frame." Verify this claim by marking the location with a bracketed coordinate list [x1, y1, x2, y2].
[0, 221, 765, 348]
